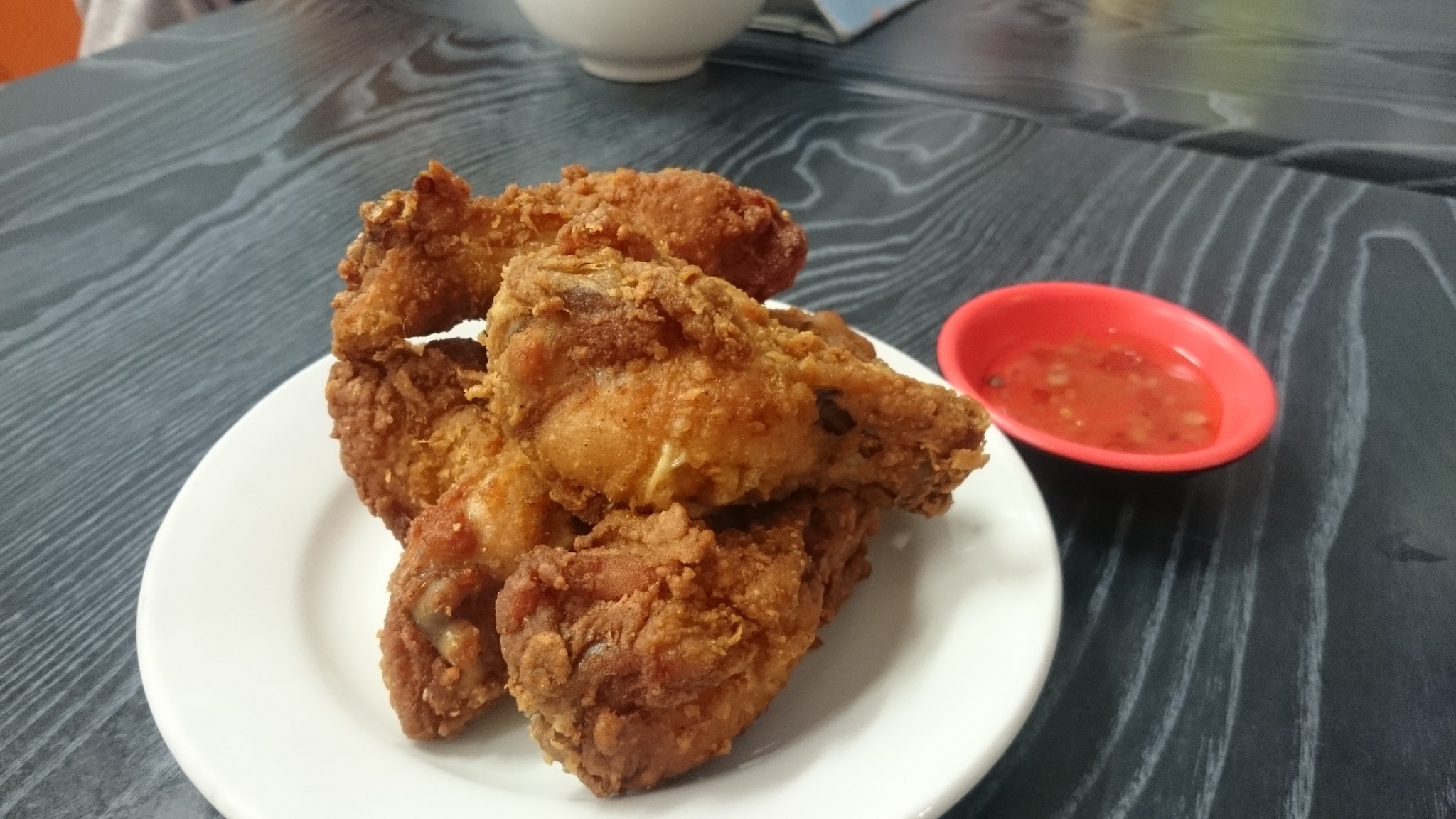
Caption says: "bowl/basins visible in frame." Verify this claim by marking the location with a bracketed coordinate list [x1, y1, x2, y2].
[513, 0, 764, 83]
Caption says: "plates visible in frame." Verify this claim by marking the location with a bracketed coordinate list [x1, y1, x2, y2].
[936, 282, 1278, 470]
[137, 298, 1064, 817]
